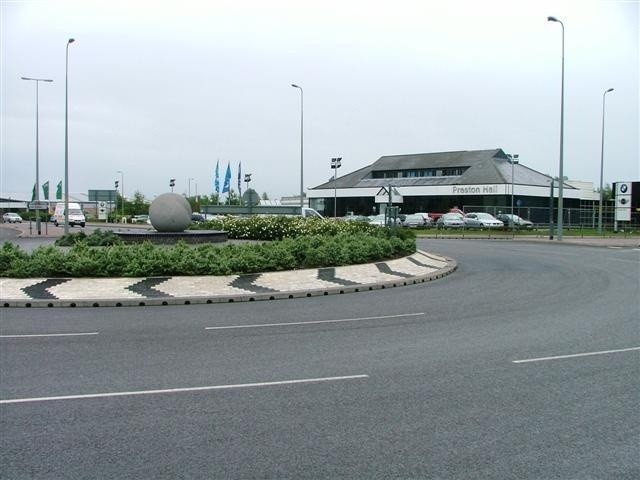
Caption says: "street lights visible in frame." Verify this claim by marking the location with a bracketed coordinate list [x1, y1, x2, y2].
[508, 153, 519, 230]
[170, 178, 176, 191]
[62, 37, 76, 233]
[597, 87, 615, 231]
[114, 170, 125, 222]
[548, 16, 566, 240]
[292, 84, 304, 214]
[330, 157, 344, 221]
[19, 77, 54, 230]
[245, 173, 253, 191]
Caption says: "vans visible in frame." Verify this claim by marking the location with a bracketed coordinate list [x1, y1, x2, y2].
[55, 202, 86, 227]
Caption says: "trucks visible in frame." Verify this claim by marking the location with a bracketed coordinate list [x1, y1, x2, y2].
[428, 209, 465, 220]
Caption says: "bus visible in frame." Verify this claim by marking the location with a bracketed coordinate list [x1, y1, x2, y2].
[200, 205, 325, 220]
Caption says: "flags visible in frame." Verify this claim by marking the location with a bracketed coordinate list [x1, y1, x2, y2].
[221, 162, 232, 193]
[213, 160, 219, 192]
[56, 180, 62, 200]
[41, 180, 48, 200]
[237, 161, 242, 196]
[31, 183, 36, 204]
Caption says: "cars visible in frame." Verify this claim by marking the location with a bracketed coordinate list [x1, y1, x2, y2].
[333, 211, 431, 232]
[438, 214, 464, 231]
[464, 212, 502, 231]
[51, 215, 57, 222]
[498, 212, 534, 231]
[3, 213, 24, 224]
[132, 215, 148, 222]
